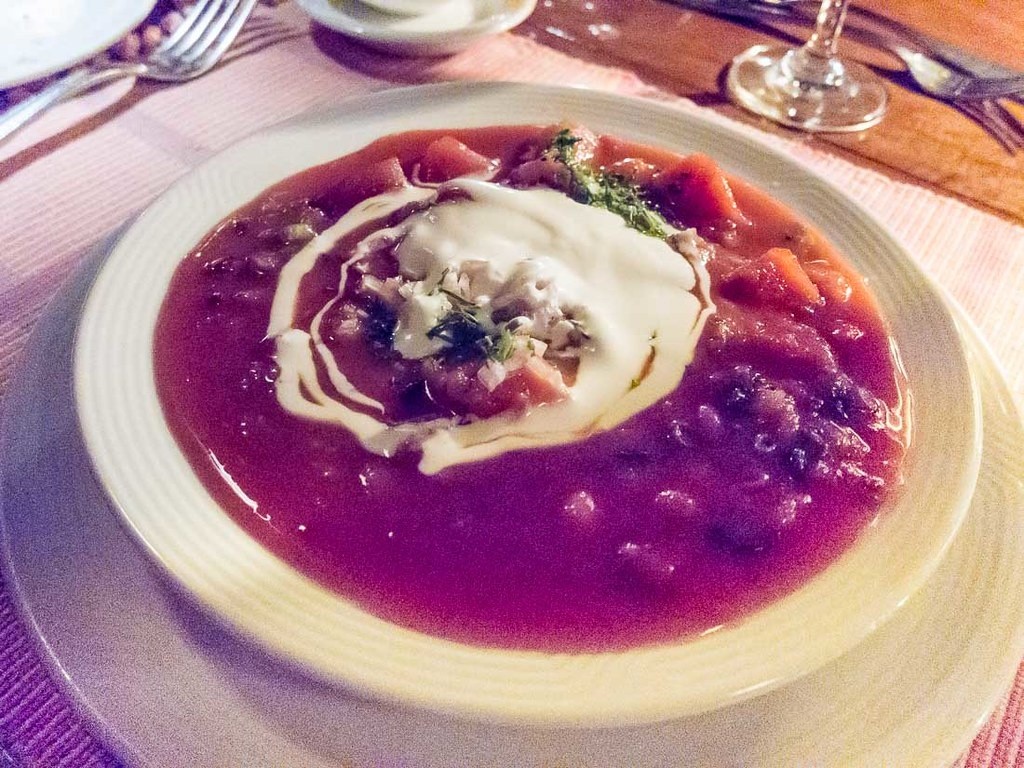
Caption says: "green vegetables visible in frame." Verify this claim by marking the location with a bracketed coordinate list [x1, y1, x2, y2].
[424, 265, 539, 370]
[541, 125, 674, 245]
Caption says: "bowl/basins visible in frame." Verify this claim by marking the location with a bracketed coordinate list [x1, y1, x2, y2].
[297, 1, 539, 59]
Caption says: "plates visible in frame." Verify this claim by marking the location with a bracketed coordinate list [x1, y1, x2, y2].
[57, 82, 982, 726]
[0, 194, 1023, 766]
[0, 1, 162, 90]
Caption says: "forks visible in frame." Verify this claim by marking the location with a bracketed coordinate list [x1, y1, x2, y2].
[0, 0, 263, 149]
[679, 0, 1024, 101]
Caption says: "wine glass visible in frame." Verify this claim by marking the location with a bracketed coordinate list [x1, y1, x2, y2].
[725, 1, 892, 133]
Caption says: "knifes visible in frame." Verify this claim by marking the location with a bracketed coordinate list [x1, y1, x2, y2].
[788, 0, 1023, 106]
